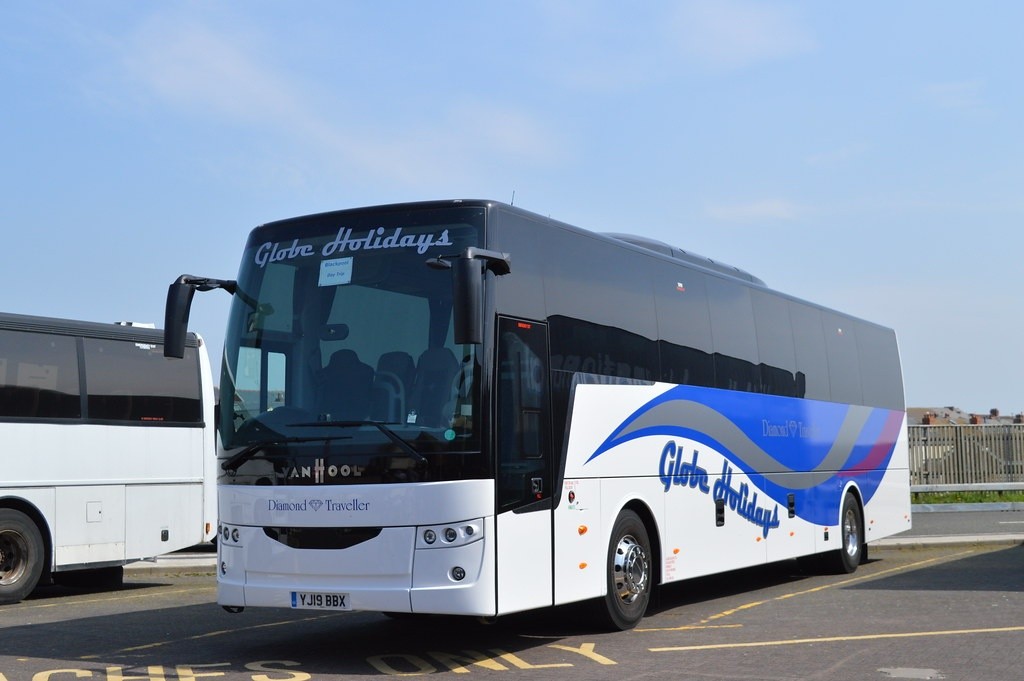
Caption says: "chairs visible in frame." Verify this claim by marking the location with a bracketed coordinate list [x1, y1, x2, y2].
[309, 347, 458, 427]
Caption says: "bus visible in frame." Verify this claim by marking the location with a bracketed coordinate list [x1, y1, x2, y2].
[0, 312, 220, 616]
[164, 198, 915, 629]
